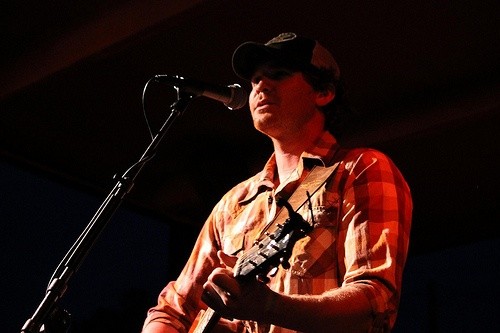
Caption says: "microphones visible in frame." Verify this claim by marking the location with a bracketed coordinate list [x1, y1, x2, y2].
[153, 75, 247, 110]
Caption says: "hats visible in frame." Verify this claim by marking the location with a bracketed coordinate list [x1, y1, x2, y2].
[231, 32, 340, 90]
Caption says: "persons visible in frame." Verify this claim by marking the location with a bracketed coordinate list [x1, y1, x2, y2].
[139, 29, 411, 333]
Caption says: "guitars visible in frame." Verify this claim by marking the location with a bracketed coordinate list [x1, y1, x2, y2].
[186, 211, 311, 332]
